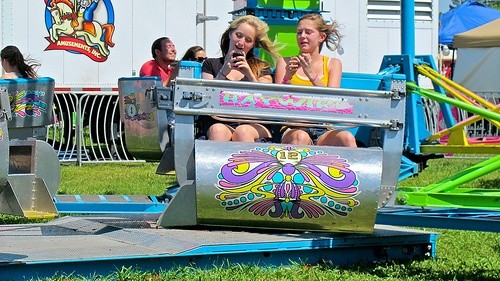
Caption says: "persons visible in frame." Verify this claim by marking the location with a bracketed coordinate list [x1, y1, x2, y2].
[140, 37, 207, 144]
[274, 14, 358, 148]
[201, 15, 274, 142]
[0, 45, 41, 79]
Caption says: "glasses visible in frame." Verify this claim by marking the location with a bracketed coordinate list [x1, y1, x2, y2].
[193, 57, 208, 63]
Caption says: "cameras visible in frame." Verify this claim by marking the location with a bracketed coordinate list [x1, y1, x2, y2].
[233, 51, 244, 67]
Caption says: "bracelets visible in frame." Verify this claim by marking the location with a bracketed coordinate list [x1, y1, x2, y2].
[219, 70, 227, 76]
[281, 75, 292, 85]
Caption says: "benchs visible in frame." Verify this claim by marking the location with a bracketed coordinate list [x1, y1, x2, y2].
[118, 76, 169, 158]
[0, 77, 61, 220]
[157, 60, 406, 234]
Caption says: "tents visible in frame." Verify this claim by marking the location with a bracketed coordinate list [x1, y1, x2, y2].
[439, 2, 500, 99]
